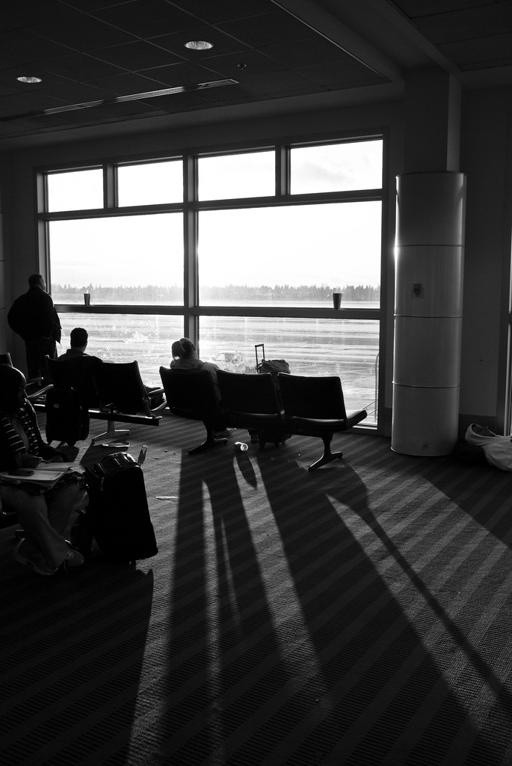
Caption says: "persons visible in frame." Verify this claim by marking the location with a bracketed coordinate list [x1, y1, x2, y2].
[0, 364, 85, 579]
[58, 327, 103, 361]
[7, 273, 62, 402]
[171, 337, 239, 438]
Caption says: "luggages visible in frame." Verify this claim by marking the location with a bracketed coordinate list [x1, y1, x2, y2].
[98, 452, 161, 560]
[248, 344, 293, 449]
[45, 383, 90, 448]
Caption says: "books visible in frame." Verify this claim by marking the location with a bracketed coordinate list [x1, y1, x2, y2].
[0, 460, 76, 491]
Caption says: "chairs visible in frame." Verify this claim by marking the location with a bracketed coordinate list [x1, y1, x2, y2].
[25, 352, 168, 435]
[160, 360, 369, 471]
